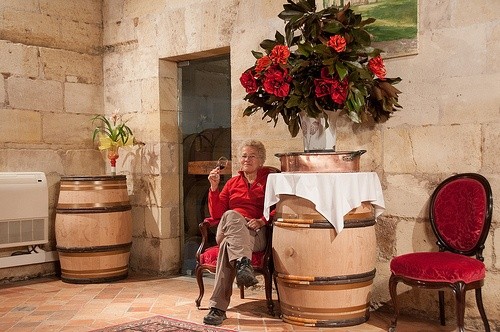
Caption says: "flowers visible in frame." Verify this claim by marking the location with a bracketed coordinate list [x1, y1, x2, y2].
[81, 109, 145, 158]
[239, 0, 402, 137]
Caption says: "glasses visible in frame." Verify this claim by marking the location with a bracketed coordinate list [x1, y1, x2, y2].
[239, 155, 262, 160]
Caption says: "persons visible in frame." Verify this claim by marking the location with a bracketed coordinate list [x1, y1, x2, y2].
[203, 141, 282, 326]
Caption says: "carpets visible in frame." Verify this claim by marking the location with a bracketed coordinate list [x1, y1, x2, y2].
[91, 316, 235, 332]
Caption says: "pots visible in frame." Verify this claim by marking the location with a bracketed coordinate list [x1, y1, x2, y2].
[273, 148, 366, 172]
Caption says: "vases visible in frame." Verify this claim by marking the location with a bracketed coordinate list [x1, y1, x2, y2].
[109, 146, 119, 175]
[299, 103, 340, 151]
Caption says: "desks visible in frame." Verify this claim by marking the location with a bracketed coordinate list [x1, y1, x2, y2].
[187, 160, 233, 229]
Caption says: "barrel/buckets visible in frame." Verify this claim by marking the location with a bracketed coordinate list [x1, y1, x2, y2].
[184, 127, 231, 160]
[267, 170, 378, 328]
[183, 173, 217, 239]
[55, 174, 132, 284]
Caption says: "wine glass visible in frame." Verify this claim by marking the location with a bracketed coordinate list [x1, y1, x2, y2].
[210, 157, 228, 182]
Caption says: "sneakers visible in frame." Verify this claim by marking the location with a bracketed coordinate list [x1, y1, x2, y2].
[235, 259, 259, 287]
[203, 307, 227, 326]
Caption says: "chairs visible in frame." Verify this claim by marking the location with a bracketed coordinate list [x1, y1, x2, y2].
[195, 166, 280, 317]
[388, 174, 492, 332]
